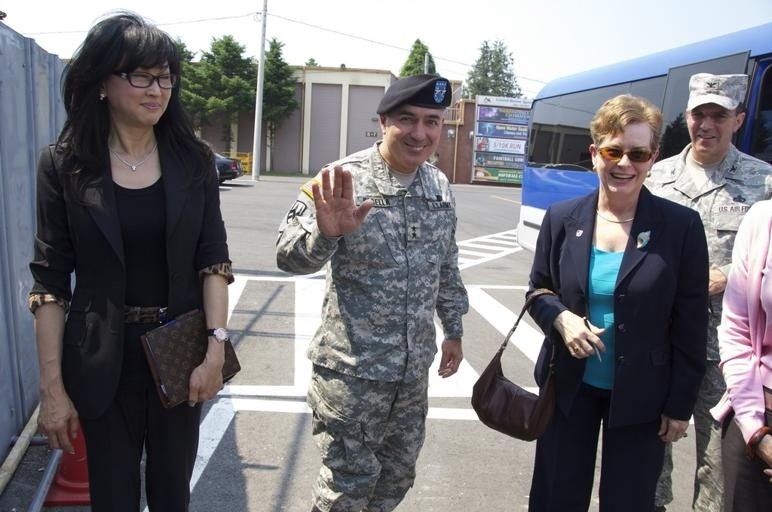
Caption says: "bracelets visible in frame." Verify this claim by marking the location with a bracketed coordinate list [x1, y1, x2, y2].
[745, 426, 771, 462]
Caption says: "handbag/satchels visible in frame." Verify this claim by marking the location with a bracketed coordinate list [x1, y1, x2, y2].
[469, 343, 564, 441]
[139, 305, 241, 409]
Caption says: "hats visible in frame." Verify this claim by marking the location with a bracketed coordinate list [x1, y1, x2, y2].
[375, 72, 452, 113]
[684, 71, 750, 112]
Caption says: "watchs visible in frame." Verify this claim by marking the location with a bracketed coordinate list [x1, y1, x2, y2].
[206, 327, 229, 343]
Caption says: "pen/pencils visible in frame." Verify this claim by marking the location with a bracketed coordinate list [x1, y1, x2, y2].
[583, 317, 602, 363]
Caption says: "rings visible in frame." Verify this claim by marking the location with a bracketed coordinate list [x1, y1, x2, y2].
[573, 346, 580, 355]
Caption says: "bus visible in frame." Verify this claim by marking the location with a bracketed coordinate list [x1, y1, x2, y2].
[516, 20, 772, 257]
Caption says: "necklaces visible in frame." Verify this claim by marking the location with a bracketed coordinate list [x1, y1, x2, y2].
[595, 208, 635, 224]
[109, 142, 158, 171]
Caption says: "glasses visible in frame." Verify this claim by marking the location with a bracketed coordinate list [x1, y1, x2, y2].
[108, 68, 179, 91]
[595, 144, 657, 164]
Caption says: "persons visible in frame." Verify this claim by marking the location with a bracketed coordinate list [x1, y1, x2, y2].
[644, 69, 771, 510]
[706, 200, 771, 510]
[523, 92, 713, 511]
[27, 8, 234, 512]
[273, 76, 472, 512]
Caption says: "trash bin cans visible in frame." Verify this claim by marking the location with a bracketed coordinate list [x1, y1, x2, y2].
[221, 152, 252, 175]
